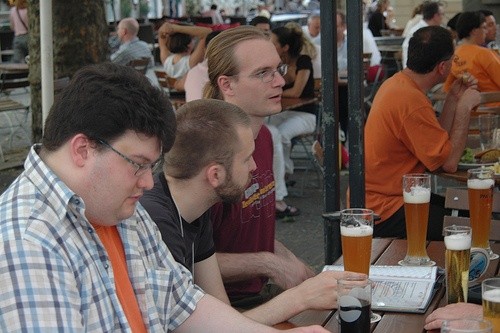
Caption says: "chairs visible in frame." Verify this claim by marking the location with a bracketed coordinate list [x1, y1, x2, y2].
[287, 78, 322, 194]
[152, 71, 185, 110]
[361, 52, 374, 108]
[128, 56, 150, 74]
[466, 91, 500, 149]
[442, 188, 500, 244]
[0, 70, 33, 161]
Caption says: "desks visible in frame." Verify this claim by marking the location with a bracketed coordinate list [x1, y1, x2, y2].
[266, 97, 318, 123]
[286, 235, 500, 333]
[429, 168, 500, 185]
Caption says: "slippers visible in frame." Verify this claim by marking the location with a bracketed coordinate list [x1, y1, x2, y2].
[276, 204, 300, 218]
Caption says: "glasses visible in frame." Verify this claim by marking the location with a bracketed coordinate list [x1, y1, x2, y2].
[93, 136, 159, 177]
[231, 63, 289, 83]
[435, 247, 489, 290]
[447, 54, 459, 62]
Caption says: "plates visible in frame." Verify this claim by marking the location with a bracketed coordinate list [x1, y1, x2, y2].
[458, 164, 497, 169]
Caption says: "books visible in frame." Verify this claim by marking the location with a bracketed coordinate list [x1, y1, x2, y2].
[320, 265, 443, 313]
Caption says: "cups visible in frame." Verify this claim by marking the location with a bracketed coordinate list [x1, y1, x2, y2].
[402, 173, 431, 264]
[340, 209, 373, 278]
[482, 278, 500, 332]
[440, 318, 492, 333]
[468, 170, 496, 255]
[337, 276, 374, 333]
[479, 115, 498, 152]
[443, 227, 472, 305]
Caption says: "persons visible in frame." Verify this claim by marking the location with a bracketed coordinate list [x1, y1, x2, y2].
[139, 100, 368, 326]
[346, 26, 482, 239]
[402, 0, 444, 69]
[111, 18, 154, 68]
[304, 0, 403, 138]
[205, 0, 270, 25]
[157, 22, 222, 101]
[200, 26, 314, 310]
[250, 16, 320, 185]
[424, 303, 500, 333]
[0, 64, 331, 333]
[449, 11, 500, 106]
[9, 0, 29, 63]
[184, 31, 301, 220]
